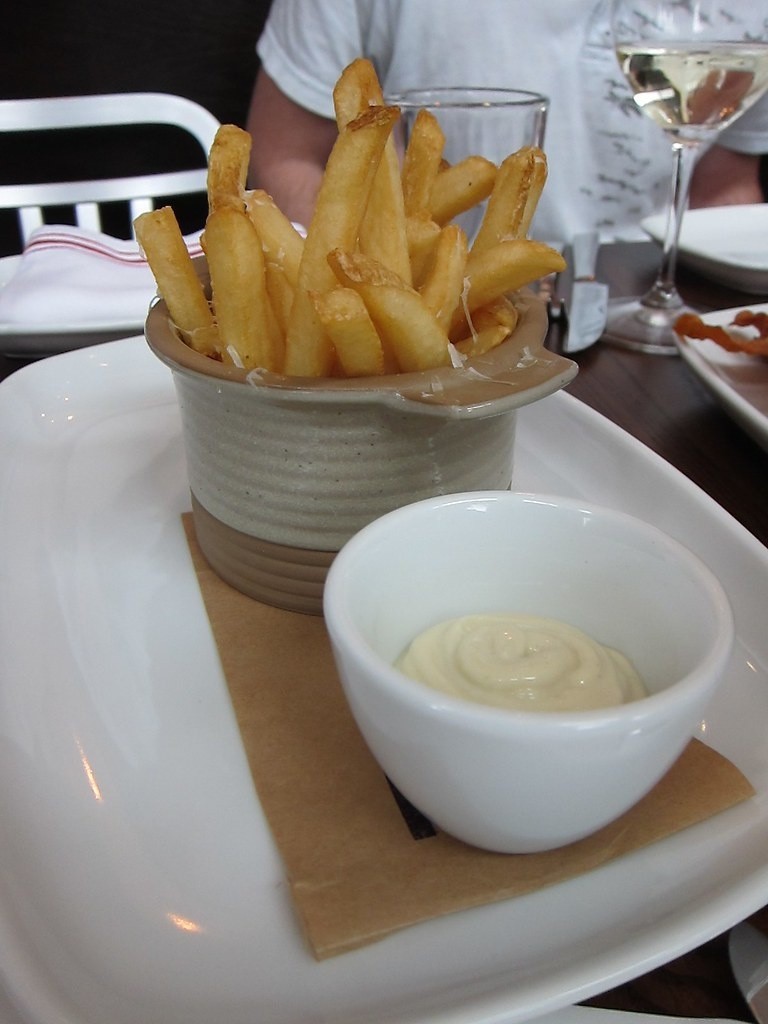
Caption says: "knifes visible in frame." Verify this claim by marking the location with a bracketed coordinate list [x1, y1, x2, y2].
[564, 233, 610, 352]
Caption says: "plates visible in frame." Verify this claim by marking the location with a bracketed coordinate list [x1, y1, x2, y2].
[639, 203, 768, 295]
[1, 256, 150, 358]
[672, 303, 768, 449]
[0, 339, 768, 1024]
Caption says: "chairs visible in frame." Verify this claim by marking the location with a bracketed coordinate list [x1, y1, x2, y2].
[0, 92, 221, 336]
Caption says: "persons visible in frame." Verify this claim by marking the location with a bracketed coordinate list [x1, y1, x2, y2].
[245, 0, 767, 235]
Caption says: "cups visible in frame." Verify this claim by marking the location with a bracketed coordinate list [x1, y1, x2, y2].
[381, 87, 551, 250]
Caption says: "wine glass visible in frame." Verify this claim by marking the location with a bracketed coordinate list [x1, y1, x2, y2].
[599, 41, 768, 354]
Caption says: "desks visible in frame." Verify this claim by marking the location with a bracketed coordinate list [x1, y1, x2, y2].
[0, 242, 768, 1024]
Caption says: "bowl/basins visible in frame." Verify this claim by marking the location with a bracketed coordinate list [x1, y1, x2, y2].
[321, 492, 735, 855]
[142, 277, 580, 616]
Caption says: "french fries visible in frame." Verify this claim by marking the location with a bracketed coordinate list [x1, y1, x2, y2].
[130, 58, 565, 381]
